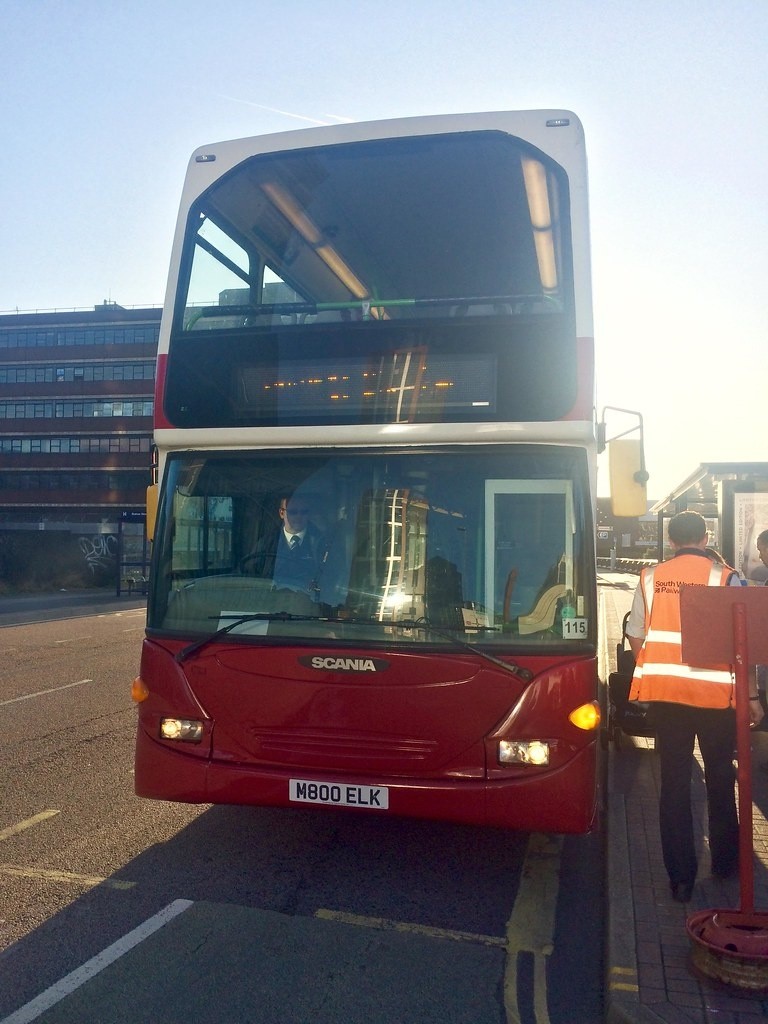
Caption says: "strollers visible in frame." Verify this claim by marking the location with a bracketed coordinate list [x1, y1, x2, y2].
[604, 610, 662, 753]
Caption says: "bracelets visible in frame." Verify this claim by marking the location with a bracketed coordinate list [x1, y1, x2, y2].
[749, 694, 760, 700]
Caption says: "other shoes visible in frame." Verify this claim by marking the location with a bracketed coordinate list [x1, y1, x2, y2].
[710, 858, 738, 881]
[671, 882, 691, 903]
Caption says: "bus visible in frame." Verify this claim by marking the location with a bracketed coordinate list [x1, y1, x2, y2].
[128, 102, 649, 843]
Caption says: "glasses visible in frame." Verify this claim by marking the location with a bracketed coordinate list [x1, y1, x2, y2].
[283, 508, 309, 515]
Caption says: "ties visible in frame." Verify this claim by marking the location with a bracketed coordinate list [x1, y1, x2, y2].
[290, 535, 301, 553]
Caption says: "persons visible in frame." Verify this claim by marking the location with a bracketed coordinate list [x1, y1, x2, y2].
[626, 509, 768, 902]
[245, 494, 345, 592]
